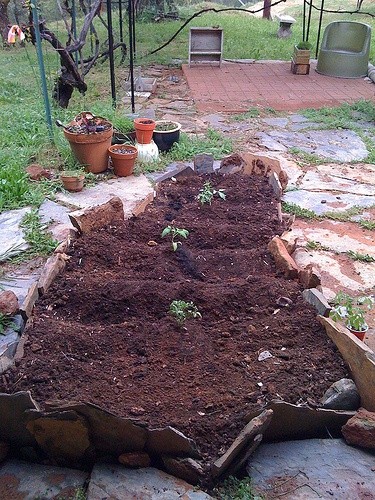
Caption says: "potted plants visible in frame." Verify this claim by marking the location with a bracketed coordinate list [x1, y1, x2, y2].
[108, 143, 140, 176]
[151, 120, 182, 154]
[327, 289, 374, 343]
[290, 40, 314, 76]
[133, 118, 156, 145]
[55, 111, 114, 173]
[58, 166, 85, 193]
[108, 116, 135, 147]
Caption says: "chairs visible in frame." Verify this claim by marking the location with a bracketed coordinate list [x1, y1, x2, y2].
[315, 19, 372, 79]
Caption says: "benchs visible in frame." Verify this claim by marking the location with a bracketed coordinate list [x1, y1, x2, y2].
[275, 13, 297, 40]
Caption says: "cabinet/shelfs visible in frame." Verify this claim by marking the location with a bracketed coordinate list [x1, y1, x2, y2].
[188, 26, 224, 69]
